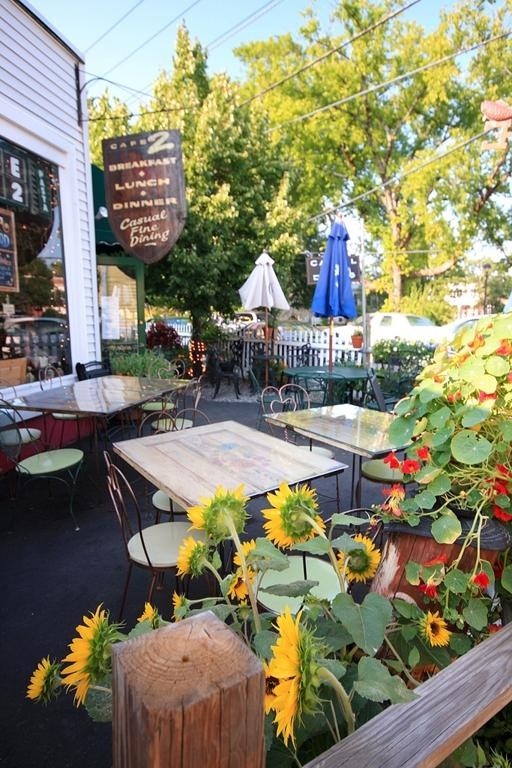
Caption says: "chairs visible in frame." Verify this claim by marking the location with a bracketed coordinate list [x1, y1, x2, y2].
[0, 353, 421, 630]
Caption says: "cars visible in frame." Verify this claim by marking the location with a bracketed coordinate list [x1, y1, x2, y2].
[146, 316, 191, 325]
[235, 312, 274, 322]
[321, 312, 512, 346]
[3, 318, 68, 334]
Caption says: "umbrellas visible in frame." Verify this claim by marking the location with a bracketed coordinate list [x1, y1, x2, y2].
[311, 211, 359, 403]
[239, 246, 291, 390]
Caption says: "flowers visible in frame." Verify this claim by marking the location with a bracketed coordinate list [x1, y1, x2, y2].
[363, 313, 509, 599]
